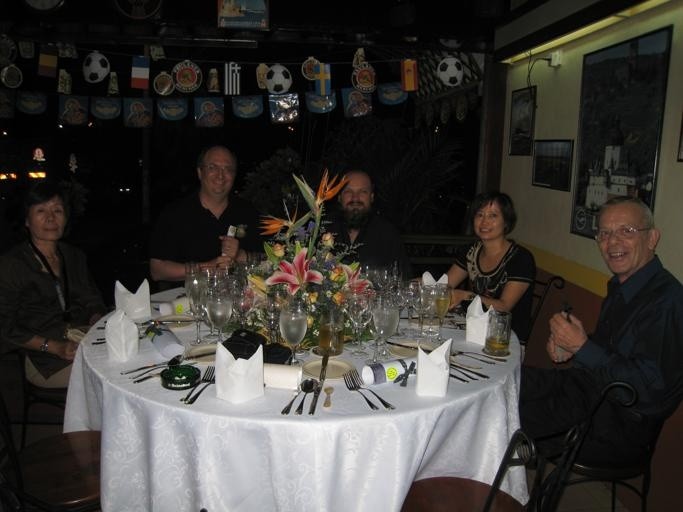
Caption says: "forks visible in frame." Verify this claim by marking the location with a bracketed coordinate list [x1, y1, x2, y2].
[342, 368, 396, 413]
[178, 366, 216, 402]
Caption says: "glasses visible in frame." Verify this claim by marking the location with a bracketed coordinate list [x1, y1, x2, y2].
[204, 164, 235, 173]
[595, 225, 650, 240]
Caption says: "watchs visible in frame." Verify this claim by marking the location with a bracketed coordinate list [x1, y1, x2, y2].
[39, 339, 49, 354]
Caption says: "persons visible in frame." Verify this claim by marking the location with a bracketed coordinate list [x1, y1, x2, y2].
[147, 142, 263, 295]
[0, 181, 115, 390]
[321, 167, 398, 267]
[408, 190, 540, 346]
[236, 137, 321, 221]
[519, 196, 682, 474]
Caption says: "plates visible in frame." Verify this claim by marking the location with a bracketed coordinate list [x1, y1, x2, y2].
[157, 315, 190, 330]
[186, 344, 216, 363]
[300, 359, 357, 381]
[385, 340, 435, 361]
[301, 355, 356, 381]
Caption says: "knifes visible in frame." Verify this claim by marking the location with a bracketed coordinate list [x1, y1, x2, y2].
[399, 360, 417, 388]
[308, 352, 329, 414]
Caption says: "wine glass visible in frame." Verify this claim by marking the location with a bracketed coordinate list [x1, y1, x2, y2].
[185, 262, 309, 364]
[347, 262, 447, 364]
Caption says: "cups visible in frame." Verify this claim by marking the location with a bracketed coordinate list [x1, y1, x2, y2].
[486, 310, 512, 356]
[320, 307, 344, 355]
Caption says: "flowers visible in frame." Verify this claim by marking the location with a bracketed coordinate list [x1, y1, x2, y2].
[217, 165, 377, 349]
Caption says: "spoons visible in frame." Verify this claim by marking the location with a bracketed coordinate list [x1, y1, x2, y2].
[292, 377, 318, 417]
[131, 352, 185, 386]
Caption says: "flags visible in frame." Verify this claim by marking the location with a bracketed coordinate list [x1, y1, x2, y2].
[314, 64, 331, 97]
[37, 44, 57, 78]
[223, 62, 242, 95]
[131, 56, 150, 90]
[400, 58, 420, 92]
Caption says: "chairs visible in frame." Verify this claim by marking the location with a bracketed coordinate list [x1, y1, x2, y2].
[0, 349, 101, 511]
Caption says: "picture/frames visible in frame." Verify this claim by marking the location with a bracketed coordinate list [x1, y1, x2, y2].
[570, 25, 671, 239]
[508, 86, 574, 191]
